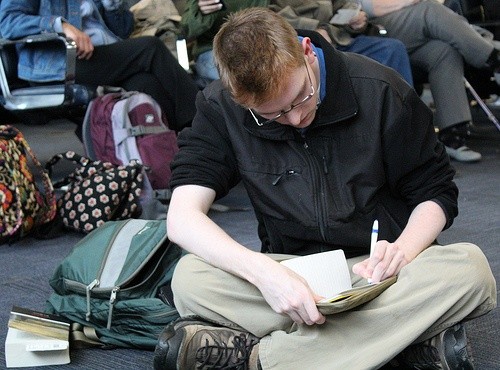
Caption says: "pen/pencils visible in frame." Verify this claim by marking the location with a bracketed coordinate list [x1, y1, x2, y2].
[367, 219, 379, 284]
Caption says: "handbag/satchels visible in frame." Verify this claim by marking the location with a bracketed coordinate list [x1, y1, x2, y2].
[43, 150, 145, 236]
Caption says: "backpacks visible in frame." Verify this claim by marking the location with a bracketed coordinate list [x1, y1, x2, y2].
[83, 85, 180, 215]
[0, 123, 57, 245]
[45, 218, 182, 347]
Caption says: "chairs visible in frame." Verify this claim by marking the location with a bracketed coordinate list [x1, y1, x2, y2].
[0, 0, 500, 147]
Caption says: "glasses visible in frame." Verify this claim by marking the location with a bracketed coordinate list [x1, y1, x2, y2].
[247, 57, 315, 127]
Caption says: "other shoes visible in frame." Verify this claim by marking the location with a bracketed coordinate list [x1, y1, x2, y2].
[446, 144, 483, 164]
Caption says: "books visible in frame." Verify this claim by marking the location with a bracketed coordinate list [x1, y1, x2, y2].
[5, 305, 70, 368]
[278, 249, 397, 314]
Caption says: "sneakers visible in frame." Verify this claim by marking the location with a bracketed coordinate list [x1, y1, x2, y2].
[398, 325, 473, 370]
[154, 314, 258, 370]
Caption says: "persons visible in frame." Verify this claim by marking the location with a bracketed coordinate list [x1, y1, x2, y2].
[154, 5, 497, 370]
[0, 0, 500, 163]
